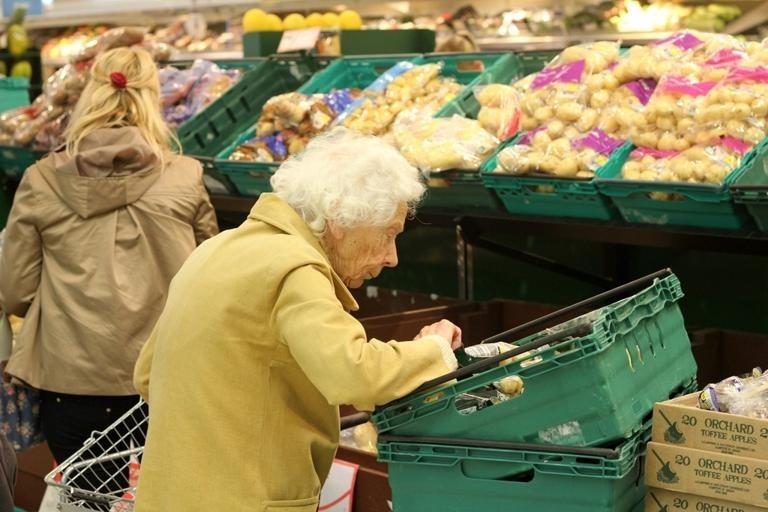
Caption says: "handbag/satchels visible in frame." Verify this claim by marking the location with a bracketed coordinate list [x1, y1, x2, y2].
[0, 376, 47, 453]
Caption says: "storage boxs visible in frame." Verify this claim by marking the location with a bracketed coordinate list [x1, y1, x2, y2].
[594, 134, 768, 229]
[417, 47, 561, 214]
[730, 146, 768, 231]
[213, 52, 419, 196]
[408, 50, 515, 119]
[479, 44, 629, 223]
[370, 266, 698, 445]
[161, 56, 269, 142]
[172, 53, 341, 195]
[375, 377, 699, 512]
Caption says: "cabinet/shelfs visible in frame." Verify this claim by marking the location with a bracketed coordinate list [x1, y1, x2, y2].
[208, 192, 768, 300]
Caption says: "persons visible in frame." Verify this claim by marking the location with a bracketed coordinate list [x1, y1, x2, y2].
[0, 46, 219, 512]
[132, 126, 462, 512]
